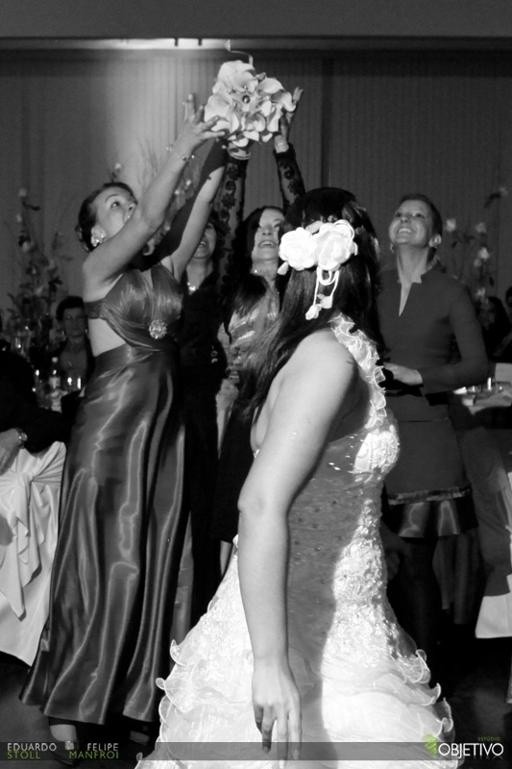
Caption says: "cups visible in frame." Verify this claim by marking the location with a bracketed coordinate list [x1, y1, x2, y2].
[475, 376, 496, 398]
[67, 377, 82, 392]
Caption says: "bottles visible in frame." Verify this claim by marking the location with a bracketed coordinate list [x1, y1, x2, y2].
[48, 358, 61, 394]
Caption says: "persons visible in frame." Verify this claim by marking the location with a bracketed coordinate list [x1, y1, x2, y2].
[2, 85, 511, 767]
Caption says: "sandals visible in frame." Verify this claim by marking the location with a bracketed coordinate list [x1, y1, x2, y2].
[47, 728, 81, 766]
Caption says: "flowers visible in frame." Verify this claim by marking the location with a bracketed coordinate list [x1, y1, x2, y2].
[201, 60, 294, 154]
